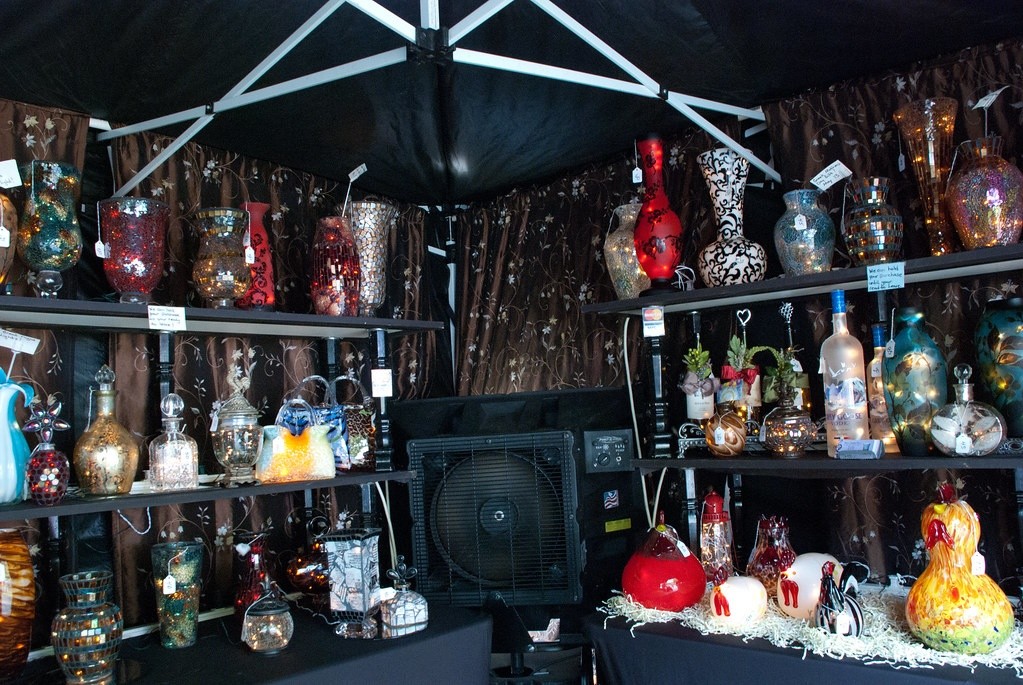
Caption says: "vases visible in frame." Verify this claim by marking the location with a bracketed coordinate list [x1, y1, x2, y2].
[841, 177, 903, 266]
[772, 188, 837, 276]
[697, 148, 768, 289]
[192, 202, 275, 310]
[51, 570, 123, 685]
[233, 529, 276, 623]
[605, 135, 685, 303]
[972, 297, 1023, 441]
[881, 307, 948, 457]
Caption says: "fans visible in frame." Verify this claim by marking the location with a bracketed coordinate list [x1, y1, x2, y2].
[408, 430, 582, 610]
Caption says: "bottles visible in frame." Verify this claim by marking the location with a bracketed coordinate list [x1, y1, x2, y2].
[894, 97, 958, 257]
[149, 393, 198, 493]
[621, 290, 1023, 655]
[696, 148, 768, 287]
[604, 203, 651, 302]
[0, 188, 17, 294]
[949, 136, 1023, 251]
[22, 402, 72, 507]
[840, 176, 903, 266]
[774, 189, 834, 276]
[633, 134, 683, 297]
[237, 203, 276, 312]
[1, 528, 35, 678]
[0, 367, 34, 507]
[73, 365, 139, 497]
[310, 217, 360, 317]
[50, 570, 123, 685]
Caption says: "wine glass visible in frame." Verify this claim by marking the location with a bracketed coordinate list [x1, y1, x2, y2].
[17, 160, 82, 299]
[96, 196, 170, 304]
[190, 207, 251, 311]
[333, 201, 397, 319]
[319, 528, 383, 639]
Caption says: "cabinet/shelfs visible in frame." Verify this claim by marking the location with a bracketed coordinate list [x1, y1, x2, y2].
[580, 244, 1023, 577]
[1, 294, 445, 664]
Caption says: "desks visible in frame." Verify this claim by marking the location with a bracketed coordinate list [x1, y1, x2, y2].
[0, 601, 491, 684]
[581, 611, 1022, 685]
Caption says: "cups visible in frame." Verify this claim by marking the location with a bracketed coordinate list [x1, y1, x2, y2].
[150, 541, 204, 649]
